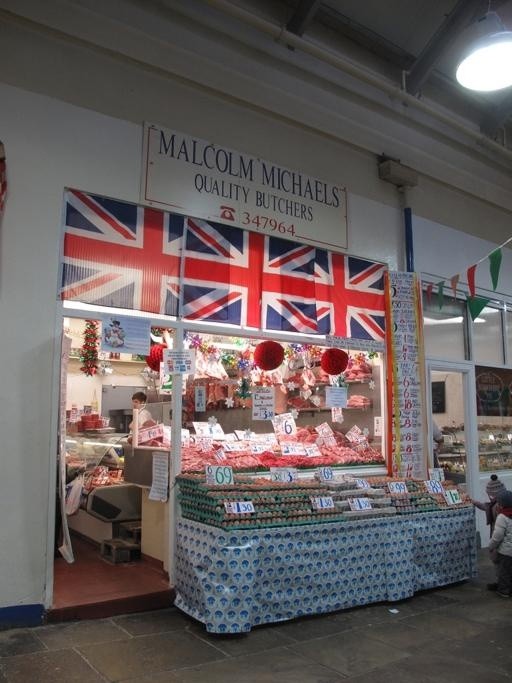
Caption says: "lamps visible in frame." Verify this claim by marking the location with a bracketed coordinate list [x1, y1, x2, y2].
[457, 10, 512, 93]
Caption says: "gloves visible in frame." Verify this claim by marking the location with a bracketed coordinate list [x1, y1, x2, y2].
[489, 542, 499, 552]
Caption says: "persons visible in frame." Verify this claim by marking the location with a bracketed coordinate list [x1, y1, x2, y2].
[469, 474, 503, 588]
[127, 392, 153, 429]
[485, 488, 511, 597]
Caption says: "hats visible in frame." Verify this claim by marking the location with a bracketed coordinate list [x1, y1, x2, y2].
[496, 490, 512, 506]
[486, 475, 506, 496]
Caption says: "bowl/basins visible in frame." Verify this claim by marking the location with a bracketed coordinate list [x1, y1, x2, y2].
[74, 414, 111, 432]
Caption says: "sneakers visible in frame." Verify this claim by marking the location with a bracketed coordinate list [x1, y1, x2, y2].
[494, 587, 510, 598]
[487, 583, 499, 591]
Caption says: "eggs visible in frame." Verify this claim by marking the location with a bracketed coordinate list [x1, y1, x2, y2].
[175, 473, 474, 528]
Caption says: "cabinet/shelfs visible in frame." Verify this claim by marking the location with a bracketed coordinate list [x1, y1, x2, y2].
[433, 430, 511, 486]
[65, 432, 144, 554]
[286, 378, 373, 417]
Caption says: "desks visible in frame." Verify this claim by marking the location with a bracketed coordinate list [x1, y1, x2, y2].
[173, 506, 479, 635]
